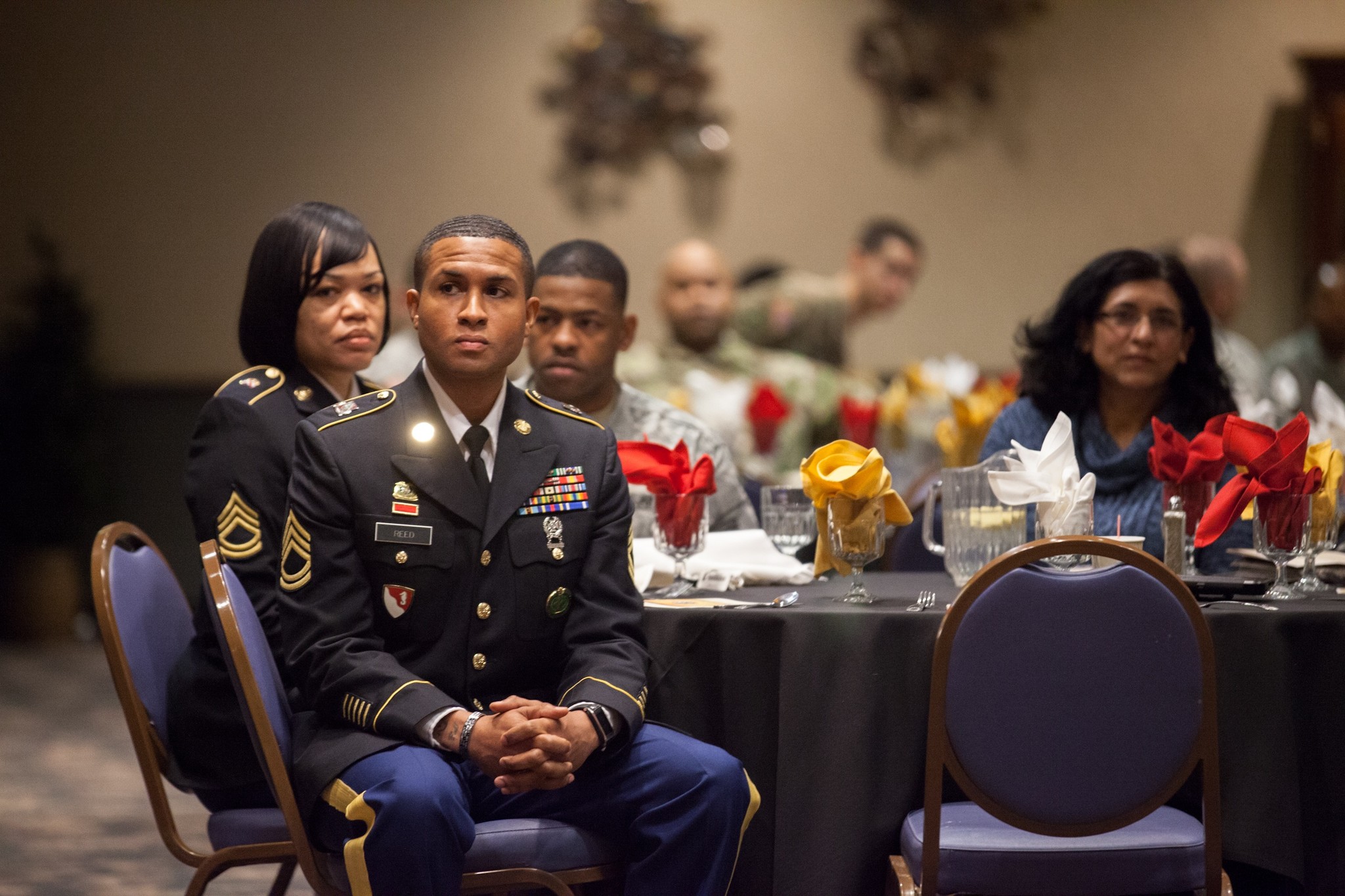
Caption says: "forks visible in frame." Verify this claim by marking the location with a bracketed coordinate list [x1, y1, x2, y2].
[906, 591, 935, 611]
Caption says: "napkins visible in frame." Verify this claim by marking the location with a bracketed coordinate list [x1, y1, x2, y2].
[746, 368, 1018, 580]
[615, 434, 716, 547]
[985, 409, 1097, 536]
[1141, 410, 1345, 553]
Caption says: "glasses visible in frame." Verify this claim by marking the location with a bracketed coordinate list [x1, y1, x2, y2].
[1092, 300, 1186, 332]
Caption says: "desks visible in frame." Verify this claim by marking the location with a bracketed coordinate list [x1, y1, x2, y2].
[643, 567, 1345, 896]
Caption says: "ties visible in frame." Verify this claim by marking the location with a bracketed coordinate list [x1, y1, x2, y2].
[462, 426, 491, 510]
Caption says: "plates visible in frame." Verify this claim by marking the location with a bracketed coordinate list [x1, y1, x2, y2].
[1180, 546, 1345, 595]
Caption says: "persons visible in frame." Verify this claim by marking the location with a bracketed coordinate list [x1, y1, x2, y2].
[1171, 231, 1344, 471]
[277, 215, 767, 896]
[612, 220, 928, 502]
[165, 201, 393, 810]
[897, 249, 1255, 585]
[513, 237, 759, 542]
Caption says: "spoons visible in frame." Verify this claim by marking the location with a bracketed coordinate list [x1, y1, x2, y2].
[713, 591, 799, 609]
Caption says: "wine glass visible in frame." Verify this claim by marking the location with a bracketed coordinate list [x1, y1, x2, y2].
[826, 497, 886, 604]
[650, 493, 712, 598]
[1160, 480, 1218, 578]
[760, 484, 817, 555]
[1250, 493, 1342, 598]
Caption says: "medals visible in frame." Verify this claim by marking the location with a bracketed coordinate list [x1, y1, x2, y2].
[545, 587, 572, 619]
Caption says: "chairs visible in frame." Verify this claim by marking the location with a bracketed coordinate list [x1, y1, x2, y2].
[884, 534, 1234, 896]
[90, 520, 624, 896]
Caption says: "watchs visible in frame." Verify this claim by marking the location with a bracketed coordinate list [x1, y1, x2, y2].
[568, 700, 617, 755]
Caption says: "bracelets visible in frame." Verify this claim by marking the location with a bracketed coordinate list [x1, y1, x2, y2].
[458, 711, 487, 762]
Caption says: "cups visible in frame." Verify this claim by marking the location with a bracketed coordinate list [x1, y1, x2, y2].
[1091, 535, 1146, 569]
[921, 466, 1027, 586]
[1035, 501, 1095, 572]
